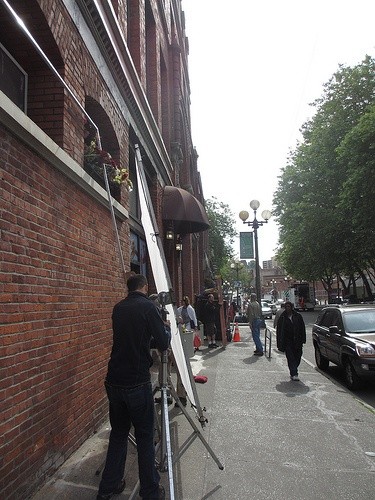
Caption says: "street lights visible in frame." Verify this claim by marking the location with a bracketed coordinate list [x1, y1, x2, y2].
[238, 199, 272, 329]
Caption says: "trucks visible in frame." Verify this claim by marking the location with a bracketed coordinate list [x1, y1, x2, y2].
[288, 282, 316, 312]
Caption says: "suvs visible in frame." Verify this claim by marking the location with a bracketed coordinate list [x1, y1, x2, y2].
[312, 304, 375, 389]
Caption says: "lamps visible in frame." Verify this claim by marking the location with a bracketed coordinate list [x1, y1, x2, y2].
[166, 224, 174, 240]
[176, 238, 183, 256]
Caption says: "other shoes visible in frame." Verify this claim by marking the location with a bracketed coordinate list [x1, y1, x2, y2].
[96, 478, 125, 500]
[253, 351, 263, 356]
[142, 486, 165, 500]
[175, 398, 187, 407]
[208, 344, 219, 348]
[291, 374, 299, 381]
[155, 396, 173, 404]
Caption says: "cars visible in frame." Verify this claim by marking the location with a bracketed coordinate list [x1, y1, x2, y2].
[244, 296, 285, 320]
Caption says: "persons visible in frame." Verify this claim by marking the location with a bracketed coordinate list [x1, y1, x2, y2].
[148, 290, 206, 408]
[202, 294, 224, 348]
[96, 275, 172, 500]
[300, 297, 305, 311]
[215, 300, 237, 340]
[274, 302, 285, 329]
[247, 293, 266, 356]
[276, 302, 306, 381]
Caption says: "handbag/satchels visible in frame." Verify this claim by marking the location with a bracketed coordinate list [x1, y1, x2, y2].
[193, 329, 201, 346]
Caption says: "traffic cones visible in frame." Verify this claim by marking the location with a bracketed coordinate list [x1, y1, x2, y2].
[233, 321, 241, 342]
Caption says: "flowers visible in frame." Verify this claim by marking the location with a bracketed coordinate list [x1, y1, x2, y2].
[84, 141, 134, 192]
